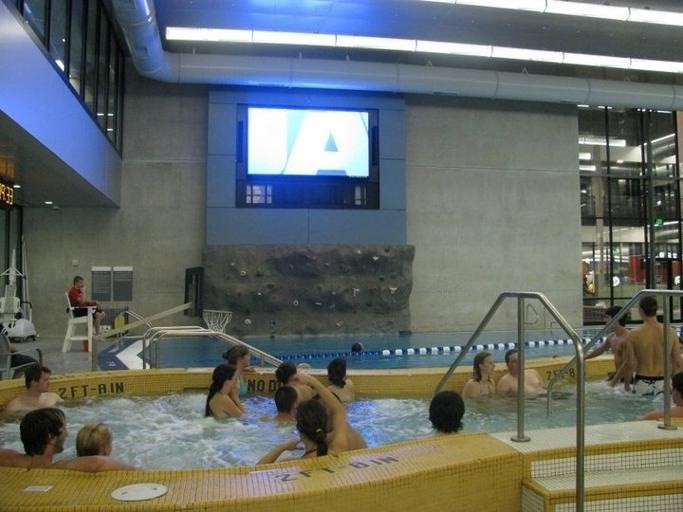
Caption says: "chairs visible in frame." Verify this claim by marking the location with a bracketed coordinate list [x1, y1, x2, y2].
[0, 333, 43, 380]
[60, 292, 96, 353]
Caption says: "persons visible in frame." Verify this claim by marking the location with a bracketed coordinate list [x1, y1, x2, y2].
[0, 323, 39, 379]
[462, 350, 496, 395]
[203, 363, 246, 420]
[636, 372, 682, 422]
[74, 421, 111, 457]
[253, 373, 348, 464]
[68, 275, 105, 336]
[583, 305, 637, 385]
[309, 390, 369, 451]
[221, 344, 255, 399]
[0, 366, 65, 418]
[258, 385, 298, 426]
[0, 407, 139, 474]
[604, 294, 682, 391]
[497, 349, 545, 398]
[426, 390, 466, 435]
[273, 360, 314, 405]
[325, 357, 355, 407]
